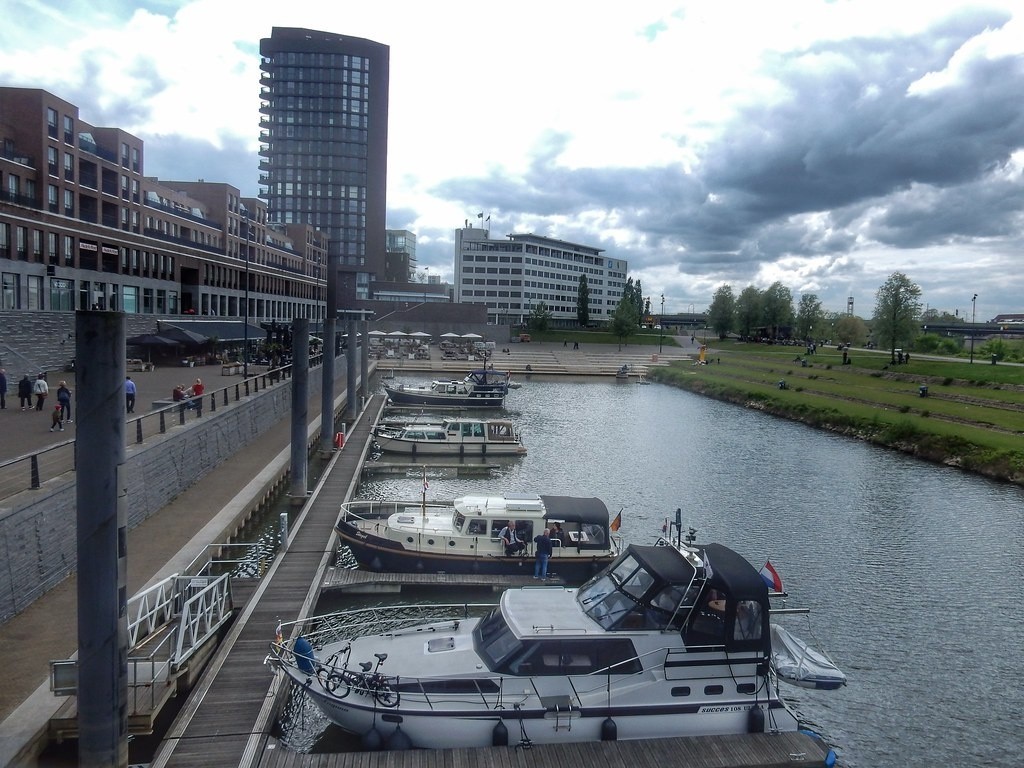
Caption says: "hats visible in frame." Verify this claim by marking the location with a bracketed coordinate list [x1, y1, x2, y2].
[59, 380, 67, 386]
[54, 405, 62, 410]
[38, 373, 46, 378]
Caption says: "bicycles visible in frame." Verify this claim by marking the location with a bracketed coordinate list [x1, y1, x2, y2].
[317, 636, 402, 709]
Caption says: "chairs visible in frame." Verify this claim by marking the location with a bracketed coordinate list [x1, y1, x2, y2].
[126, 359, 152, 372]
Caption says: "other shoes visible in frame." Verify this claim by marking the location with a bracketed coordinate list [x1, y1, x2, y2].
[28, 404, 36, 410]
[131, 408, 134, 412]
[67, 419, 74, 423]
[127, 408, 130, 413]
[61, 420, 66, 424]
[533, 576, 539, 579]
[60, 428, 65, 432]
[50, 428, 54, 432]
[20, 406, 25, 412]
[541, 578, 546, 581]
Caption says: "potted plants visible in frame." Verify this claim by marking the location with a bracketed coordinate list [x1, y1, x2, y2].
[263, 344, 283, 379]
[208, 335, 217, 365]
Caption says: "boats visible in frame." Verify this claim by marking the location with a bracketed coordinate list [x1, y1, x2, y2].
[331, 464, 625, 584]
[375, 417, 529, 458]
[383, 363, 511, 409]
[769, 622, 848, 690]
[259, 507, 848, 747]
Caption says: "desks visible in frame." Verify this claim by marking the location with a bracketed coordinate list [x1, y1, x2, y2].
[569, 531, 590, 542]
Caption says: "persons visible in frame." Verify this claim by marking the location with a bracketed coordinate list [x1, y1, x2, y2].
[533, 522, 565, 580]
[309, 347, 319, 355]
[216, 348, 240, 364]
[173, 378, 204, 410]
[810, 343, 844, 354]
[890, 353, 910, 366]
[0, 369, 74, 431]
[691, 335, 694, 344]
[498, 521, 525, 557]
[564, 338, 578, 349]
[125, 376, 135, 413]
[652, 586, 682, 624]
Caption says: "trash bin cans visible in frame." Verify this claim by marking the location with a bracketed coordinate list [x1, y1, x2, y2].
[652, 354, 658, 362]
[190, 362, 194, 367]
[919, 386, 928, 398]
[992, 354, 997, 364]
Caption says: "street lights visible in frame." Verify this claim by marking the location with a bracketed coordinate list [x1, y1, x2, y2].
[969, 292, 978, 363]
[659, 293, 666, 355]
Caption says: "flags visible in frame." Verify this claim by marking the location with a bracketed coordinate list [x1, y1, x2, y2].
[421, 474, 428, 493]
[760, 561, 782, 592]
[611, 512, 621, 531]
[704, 552, 713, 579]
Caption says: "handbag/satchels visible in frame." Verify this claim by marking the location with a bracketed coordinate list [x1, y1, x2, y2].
[42, 392, 47, 396]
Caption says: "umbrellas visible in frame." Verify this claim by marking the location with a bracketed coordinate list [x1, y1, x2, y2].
[308, 335, 324, 345]
[439, 333, 483, 338]
[368, 330, 432, 339]
[126, 325, 207, 361]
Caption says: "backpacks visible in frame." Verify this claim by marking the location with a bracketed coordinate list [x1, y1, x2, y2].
[59, 390, 70, 403]
